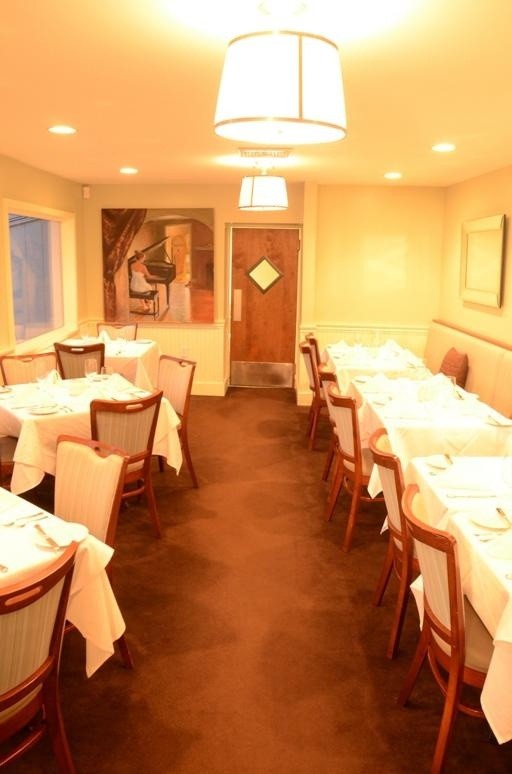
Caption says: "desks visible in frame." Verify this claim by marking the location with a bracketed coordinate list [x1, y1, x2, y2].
[195, 245, 213, 287]
[47, 337, 160, 393]
[1, 374, 182, 496]
[1, 488, 127, 680]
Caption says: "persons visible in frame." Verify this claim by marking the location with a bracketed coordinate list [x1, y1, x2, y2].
[130, 250, 153, 311]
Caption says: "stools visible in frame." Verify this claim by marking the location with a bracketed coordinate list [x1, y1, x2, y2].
[129, 289, 160, 321]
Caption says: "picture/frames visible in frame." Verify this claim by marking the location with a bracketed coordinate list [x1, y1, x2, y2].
[458, 211, 505, 309]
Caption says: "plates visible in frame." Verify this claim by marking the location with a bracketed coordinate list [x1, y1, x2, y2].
[76, 334, 134, 358]
[424, 452, 512, 581]
[1, 369, 151, 417]
[136, 338, 153, 344]
[26, 521, 89, 547]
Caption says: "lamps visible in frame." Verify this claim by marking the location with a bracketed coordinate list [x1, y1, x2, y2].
[237, 146, 290, 212]
[214, 26, 349, 150]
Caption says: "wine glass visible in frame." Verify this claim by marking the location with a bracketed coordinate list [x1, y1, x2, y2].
[84, 360, 97, 387]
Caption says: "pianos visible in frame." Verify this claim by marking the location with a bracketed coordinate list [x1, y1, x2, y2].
[128, 236, 176, 304]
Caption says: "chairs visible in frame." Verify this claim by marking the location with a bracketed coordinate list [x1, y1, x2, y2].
[90, 392, 167, 532]
[155, 354, 197, 489]
[53, 433, 139, 675]
[2, 352, 63, 386]
[299, 330, 512, 773]
[54, 342, 108, 378]
[1, 540, 83, 773]
[96, 323, 140, 341]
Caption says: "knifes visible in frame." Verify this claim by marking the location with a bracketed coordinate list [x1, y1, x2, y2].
[32, 524, 60, 552]
